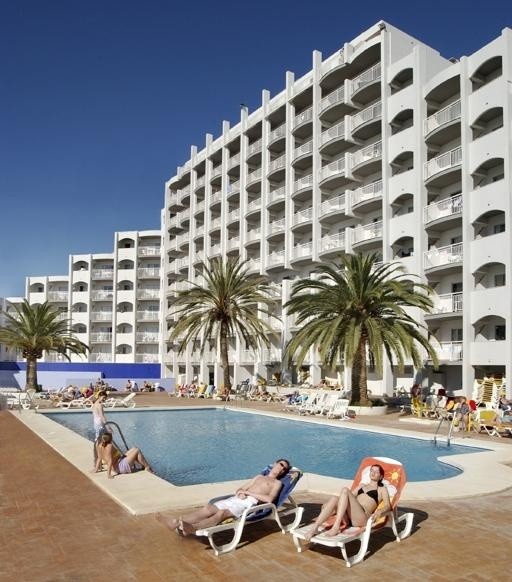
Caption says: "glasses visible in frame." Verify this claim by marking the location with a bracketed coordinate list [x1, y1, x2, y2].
[279, 462, 287, 472]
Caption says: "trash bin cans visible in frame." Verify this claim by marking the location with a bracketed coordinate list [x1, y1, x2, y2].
[38, 385, 42, 392]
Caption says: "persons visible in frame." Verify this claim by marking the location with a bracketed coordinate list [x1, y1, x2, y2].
[303, 463, 391, 542]
[94, 432, 159, 480]
[93, 390, 113, 444]
[41, 377, 153, 406]
[156, 458, 290, 537]
[409, 381, 511, 436]
[175, 378, 328, 405]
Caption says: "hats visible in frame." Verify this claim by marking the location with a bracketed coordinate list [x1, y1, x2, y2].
[447, 392, 455, 398]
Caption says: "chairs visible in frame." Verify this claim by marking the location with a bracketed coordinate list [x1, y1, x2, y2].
[174, 456, 414, 568]
